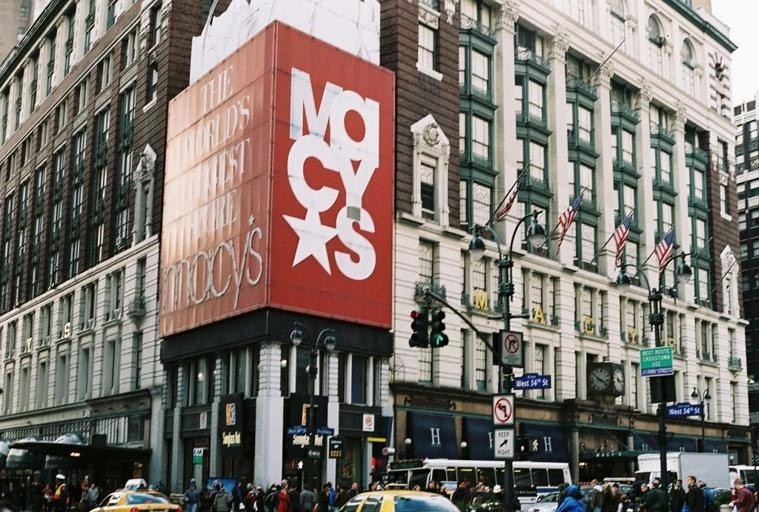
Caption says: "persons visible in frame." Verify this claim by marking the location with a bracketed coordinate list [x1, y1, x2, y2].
[728, 478, 756, 512]
[412, 480, 522, 512]
[1, 471, 99, 512]
[158, 480, 170, 499]
[368, 481, 394, 491]
[554, 476, 715, 512]
[184, 478, 358, 512]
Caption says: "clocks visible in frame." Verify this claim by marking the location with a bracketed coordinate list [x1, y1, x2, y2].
[587, 362, 625, 395]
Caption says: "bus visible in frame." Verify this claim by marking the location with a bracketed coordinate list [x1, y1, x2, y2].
[386, 455, 573, 512]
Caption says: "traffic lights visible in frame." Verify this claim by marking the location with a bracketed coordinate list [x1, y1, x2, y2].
[407, 310, 449, 349]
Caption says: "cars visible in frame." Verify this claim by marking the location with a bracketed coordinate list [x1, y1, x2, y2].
[528, 491, 560, 511]
[90, 477, 184, 512]
[337, 489, 462, 512]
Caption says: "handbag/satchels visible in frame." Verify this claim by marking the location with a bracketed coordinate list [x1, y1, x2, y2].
[247, 491, 257, 504]
[265, 493, 278, 509]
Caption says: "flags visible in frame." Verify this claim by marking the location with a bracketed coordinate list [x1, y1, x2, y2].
[613, 208, 635, 272]
[694, 236, 713, 271]
[655, 224, 676, 273]
[496, 167, 529, 222]
[555, 187, 587, 255]
[725, 260, 738, 292]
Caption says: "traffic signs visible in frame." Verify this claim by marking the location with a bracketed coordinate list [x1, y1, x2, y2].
[665, 402, 706, 417]
[285, 423, 335, 438]
[512, 371, 553, 391]
[639, 345, 674, 378]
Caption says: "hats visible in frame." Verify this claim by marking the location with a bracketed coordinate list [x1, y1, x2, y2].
[568, 485, 579, 495]
[213, 479, 223, 490]
[493, 485, 501, 493]
[55, 474, 66, 482]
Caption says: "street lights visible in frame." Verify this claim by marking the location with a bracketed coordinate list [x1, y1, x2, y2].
[467, 207, 551, 507]
[614, 252, 694, 505]
[690, 384, 721, 451]
[288, 320, 338, 507]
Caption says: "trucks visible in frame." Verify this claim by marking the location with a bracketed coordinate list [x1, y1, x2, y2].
[634, 451, 731, 496]
